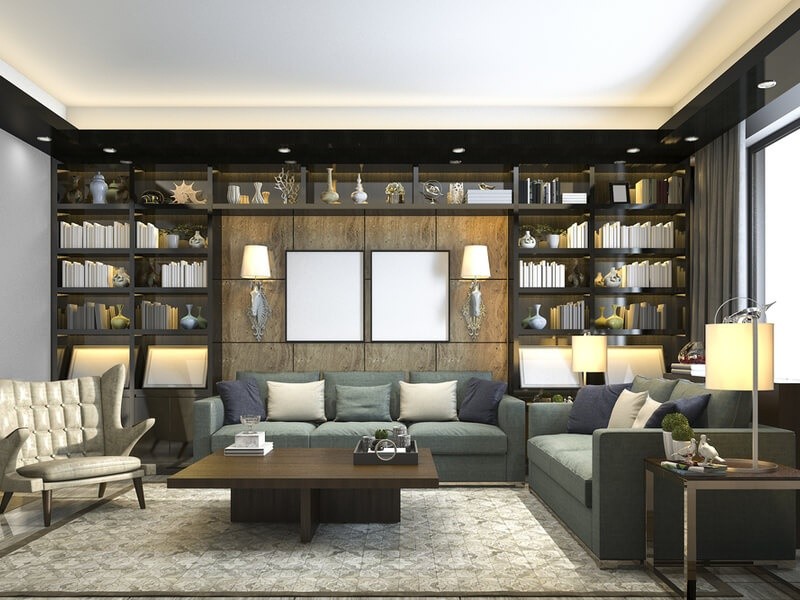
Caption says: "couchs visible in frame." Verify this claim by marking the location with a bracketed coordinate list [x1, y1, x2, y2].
[193, 371, 527, 482]
[528, 399, 796, 568]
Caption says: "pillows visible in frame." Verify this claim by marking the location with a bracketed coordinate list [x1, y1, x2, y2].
[215, 369, 509, 426]
[567, 375, 710, 437]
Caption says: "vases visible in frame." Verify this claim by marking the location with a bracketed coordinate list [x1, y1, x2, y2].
[227, 168, 340, 204]
[111, 266, 208, 329]
[521, 267, 624, 331]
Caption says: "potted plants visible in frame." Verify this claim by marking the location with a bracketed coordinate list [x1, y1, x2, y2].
[161, 225, 204, 249]
[662, 413, 689, 460]
[516, 223, 567, 248]
[671, 425, 695, 461]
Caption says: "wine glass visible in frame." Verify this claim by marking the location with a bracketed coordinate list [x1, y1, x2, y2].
[240, 415, 261, 434]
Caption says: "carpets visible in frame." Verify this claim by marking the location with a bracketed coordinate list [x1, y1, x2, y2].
[0, 483, 744, 596]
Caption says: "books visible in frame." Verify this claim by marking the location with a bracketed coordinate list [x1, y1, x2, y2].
[618, 302, 666, 329]
[162, 260, 207, 287]
[60, 221, 130, 249]
[671, 363, 705, 377]
[62, 261, 117, 287]
[616, 260, 672, 287]
[519, 260, 565, 288]
[594, 221, 674, 248]
[518, 177, 587, 204]
[137, 221, 179, 248]
[58, 302, 116, 329]
[635, 177, 683, 204]
[660, 461, 728, 473]
[133, 296, 178, 329]
[567, 221, 588, 248]
[549, 301, 589, 329]
[224, 442, 274, 456]
[461, 189, 512, 204]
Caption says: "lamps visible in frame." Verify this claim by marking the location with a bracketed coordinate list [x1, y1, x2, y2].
[460, 244, 491, 342]
[705, 296, 778, 474]
[572, 335, 607, 385]
[241, 244, 271, 341]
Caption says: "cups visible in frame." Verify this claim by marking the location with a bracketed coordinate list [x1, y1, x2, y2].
[167, 235, 179, 248]
[385, 193, 406, 205]
[226, 185, 240, 205]
[549, 235, 559, 248]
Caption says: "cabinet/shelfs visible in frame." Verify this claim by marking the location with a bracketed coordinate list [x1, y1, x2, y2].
[49, 157, 217, 469]
[510, 163, 691, 401]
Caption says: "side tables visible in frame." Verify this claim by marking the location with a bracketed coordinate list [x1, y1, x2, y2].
[644, 456, 800, 600]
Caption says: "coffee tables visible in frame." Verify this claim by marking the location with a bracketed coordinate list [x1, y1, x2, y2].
[167, 448, 439, 544]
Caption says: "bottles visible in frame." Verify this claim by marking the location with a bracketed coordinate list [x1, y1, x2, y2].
[520, 231, 536, 248]
[350, 179, 368, 204]
[529, 304, 547, 329]
[180, 305, 198, 330]
[196, 306, 207, 329]
[603, 267, 621, 287]
[110, 304, 131, 329]
[321, 167, 339, 204]
[607, 304, 623, 329]
[189, 231, 206, 250]
[521, 307, 533, 329]
[106, 183, 130, 203]
[252, 183, 264, 204]
[594, 306, 608, 329]
[90, 172, 108, 205]
[594, 272, 604, 287]
[67, 175, 83, 204]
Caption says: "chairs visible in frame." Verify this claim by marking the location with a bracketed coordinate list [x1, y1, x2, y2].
[0, 364, 155, 529]
